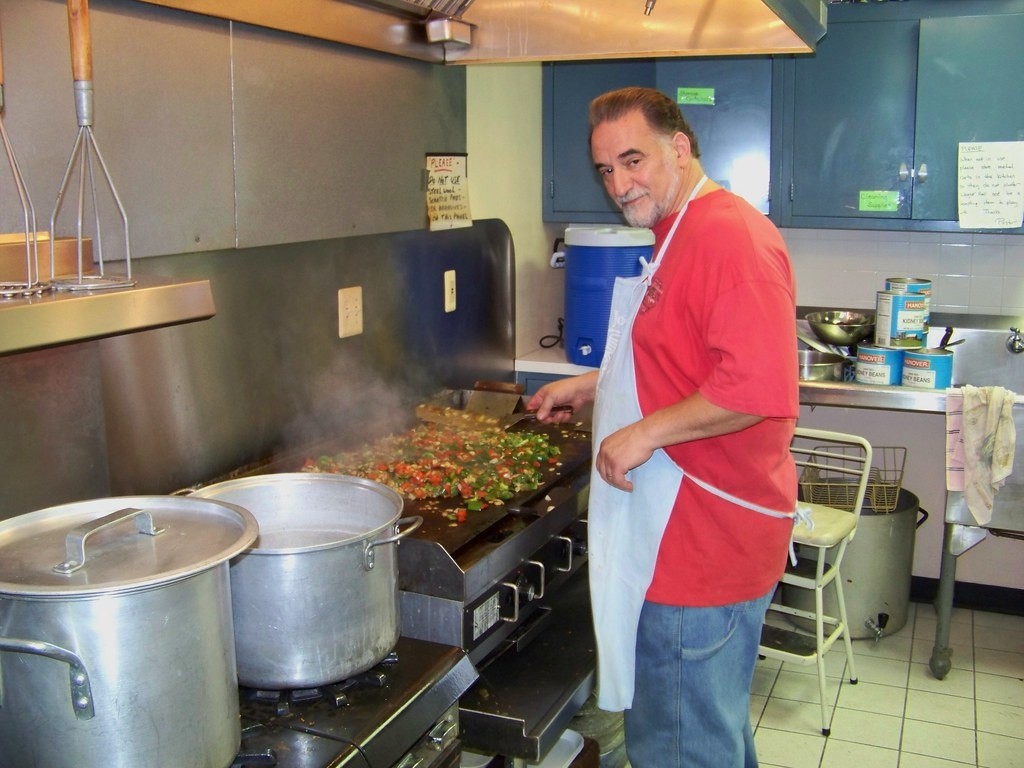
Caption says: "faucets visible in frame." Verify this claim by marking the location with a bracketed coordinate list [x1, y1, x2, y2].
[1010, 325, 1022, 353]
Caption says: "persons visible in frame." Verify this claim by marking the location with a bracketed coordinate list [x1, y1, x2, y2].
[526, 87, 798, 767]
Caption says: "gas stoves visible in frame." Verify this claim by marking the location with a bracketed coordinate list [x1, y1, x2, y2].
[218, 632, 483, 768]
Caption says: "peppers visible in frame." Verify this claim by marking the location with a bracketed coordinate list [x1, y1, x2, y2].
[299, 426, 560, 520]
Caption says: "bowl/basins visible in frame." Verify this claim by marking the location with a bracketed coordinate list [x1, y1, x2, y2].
[804, 309, 878, 345]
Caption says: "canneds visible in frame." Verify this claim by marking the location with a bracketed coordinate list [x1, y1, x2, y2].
[855, 277, 954, 390]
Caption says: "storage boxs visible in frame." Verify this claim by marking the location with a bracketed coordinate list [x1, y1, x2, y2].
[0, 232, 95, 285]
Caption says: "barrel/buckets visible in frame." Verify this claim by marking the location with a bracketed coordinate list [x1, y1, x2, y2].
[780, 483, 929, 639]
[562, 223, 656, 374]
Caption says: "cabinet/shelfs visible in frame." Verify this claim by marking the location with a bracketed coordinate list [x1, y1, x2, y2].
[542, 56, 771, 224]
[767, 2, 1024, 234]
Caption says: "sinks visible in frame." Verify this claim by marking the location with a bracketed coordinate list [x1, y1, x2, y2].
[796, 304, 1024, 418]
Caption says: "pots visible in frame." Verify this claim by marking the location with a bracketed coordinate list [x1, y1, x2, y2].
[0, 494, 260, 768]
[798, 348, 846, 382]
[168, 471, 424, 688]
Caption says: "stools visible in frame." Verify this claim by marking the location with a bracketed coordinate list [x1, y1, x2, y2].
[758, 425, 873, 736]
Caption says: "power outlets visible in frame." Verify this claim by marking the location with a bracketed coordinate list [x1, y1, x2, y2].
[338, 287, 364, 339]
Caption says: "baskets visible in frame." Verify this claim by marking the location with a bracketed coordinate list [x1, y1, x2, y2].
[798, 446, 907, 514]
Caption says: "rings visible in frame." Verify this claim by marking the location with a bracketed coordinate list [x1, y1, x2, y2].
[607, 475, 612, 478]
